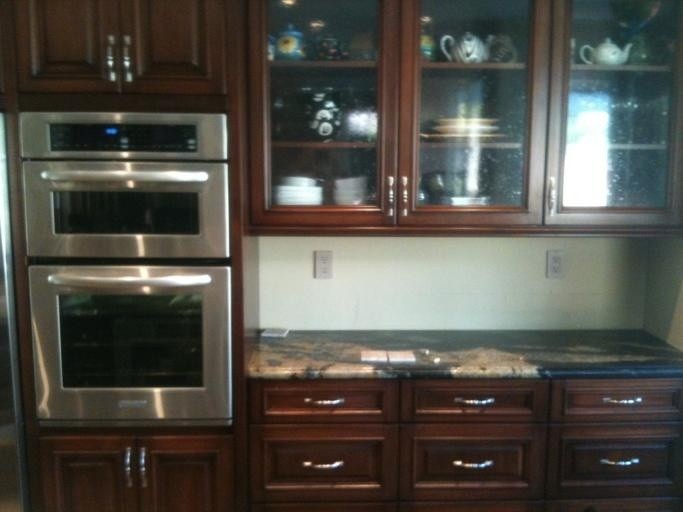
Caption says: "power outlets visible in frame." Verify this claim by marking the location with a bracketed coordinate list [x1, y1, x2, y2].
[313, 251, 332, 279]
[546, 249, 567, 277]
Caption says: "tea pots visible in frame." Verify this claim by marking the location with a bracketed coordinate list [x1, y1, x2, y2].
[577, 32, 669, 66]
[266, 22, 352, 61]
[439, 32, 496, 64]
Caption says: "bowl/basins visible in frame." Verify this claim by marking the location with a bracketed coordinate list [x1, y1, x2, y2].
[272, 172, 376, 206]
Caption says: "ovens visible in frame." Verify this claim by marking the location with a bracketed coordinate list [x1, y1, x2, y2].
[20, 108, 233, 428]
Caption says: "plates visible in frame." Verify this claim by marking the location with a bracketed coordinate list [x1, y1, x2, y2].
[422, 115, 509, 142]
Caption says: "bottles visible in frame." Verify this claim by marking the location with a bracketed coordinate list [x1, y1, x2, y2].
[418, 13, 438, 61]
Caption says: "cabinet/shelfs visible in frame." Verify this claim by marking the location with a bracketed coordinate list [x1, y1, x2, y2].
[12, 0, 246, 96]
[400, 378, 550, 512]
[550, 0, 683, 236]
[235, 379, 400, 512]
[0, 0, 16, 95]
[225, 1, 553, 236]
[26, 430, 247, 511]
[551, 377, 682, 512]
